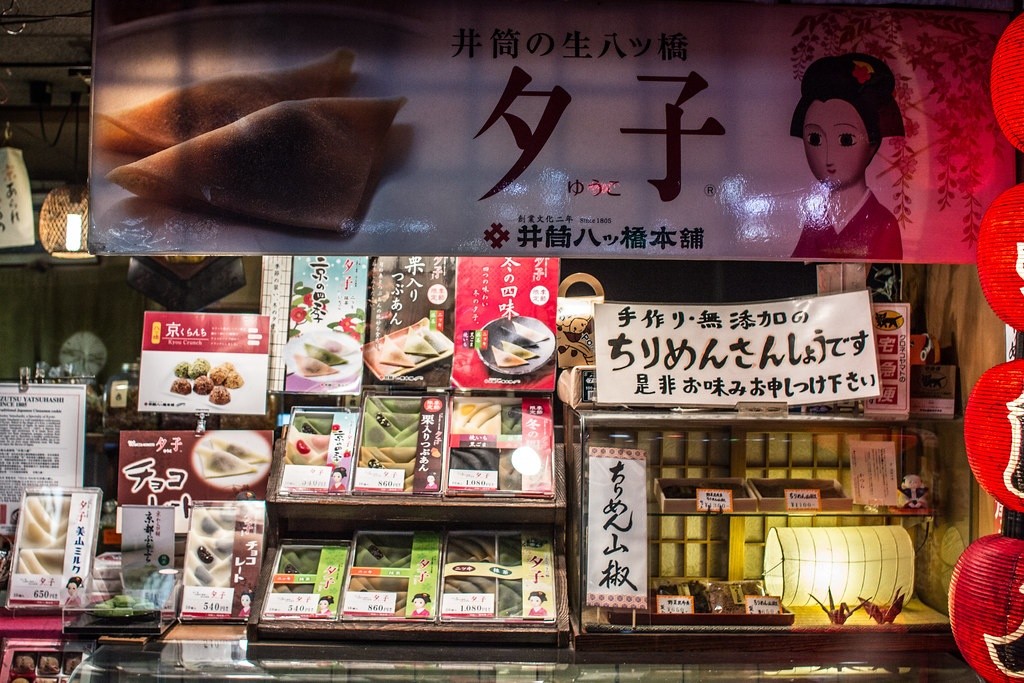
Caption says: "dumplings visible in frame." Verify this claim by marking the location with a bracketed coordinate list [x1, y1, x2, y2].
[14, 321, 551, 618]
[88, 44, 405, 234]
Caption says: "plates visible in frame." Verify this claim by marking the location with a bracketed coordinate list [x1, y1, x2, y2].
[284, 330, 362, 382]
[475, 315, 556, 376]
[363, 317, 455, 380]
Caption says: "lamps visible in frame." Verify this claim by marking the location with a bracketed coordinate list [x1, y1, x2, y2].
[39, 90, 95, 259]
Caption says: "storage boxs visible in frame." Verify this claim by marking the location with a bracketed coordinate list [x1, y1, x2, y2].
[258, 391, 557, 626]
